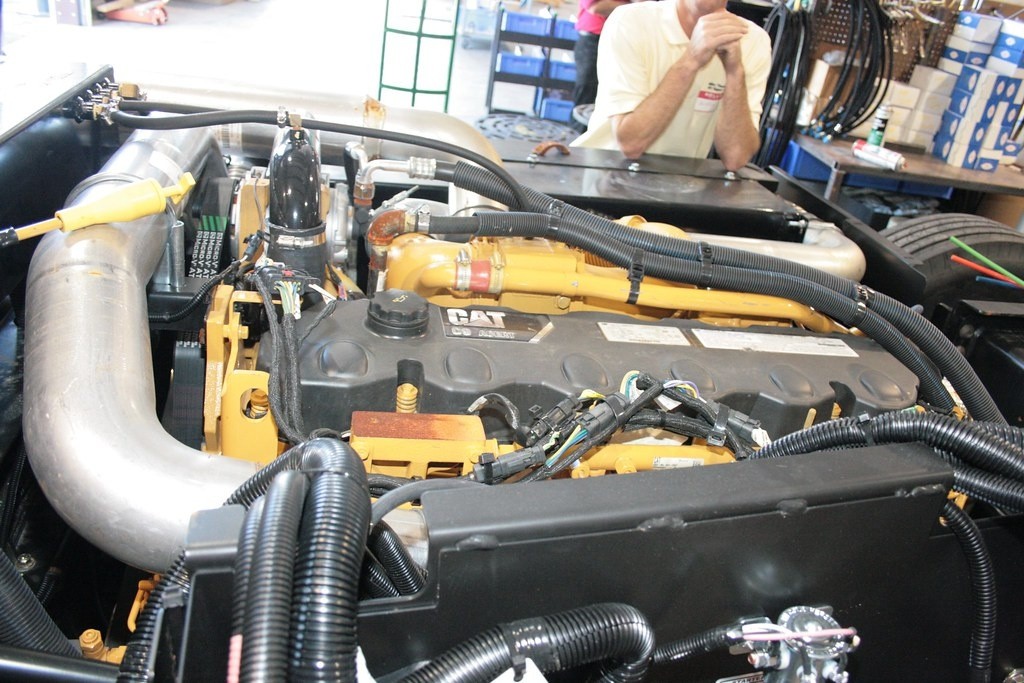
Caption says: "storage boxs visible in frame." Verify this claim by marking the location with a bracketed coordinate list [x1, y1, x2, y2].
[768, 0, 1024, 199]
[492, 11, 581, 122]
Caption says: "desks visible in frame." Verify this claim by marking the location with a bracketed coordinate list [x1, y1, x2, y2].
[791, 132, 1024, 201]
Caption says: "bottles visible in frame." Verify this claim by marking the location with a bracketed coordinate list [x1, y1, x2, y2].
[866, 100, 894, 148]
[850, 139, 909, 171]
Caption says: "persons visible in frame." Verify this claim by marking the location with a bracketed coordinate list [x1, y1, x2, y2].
[567, 0, 640, 136]
[569, 0, 772, 173]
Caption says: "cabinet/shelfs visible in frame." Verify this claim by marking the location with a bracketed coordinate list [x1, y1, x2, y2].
[485, 10, 577, 122]
